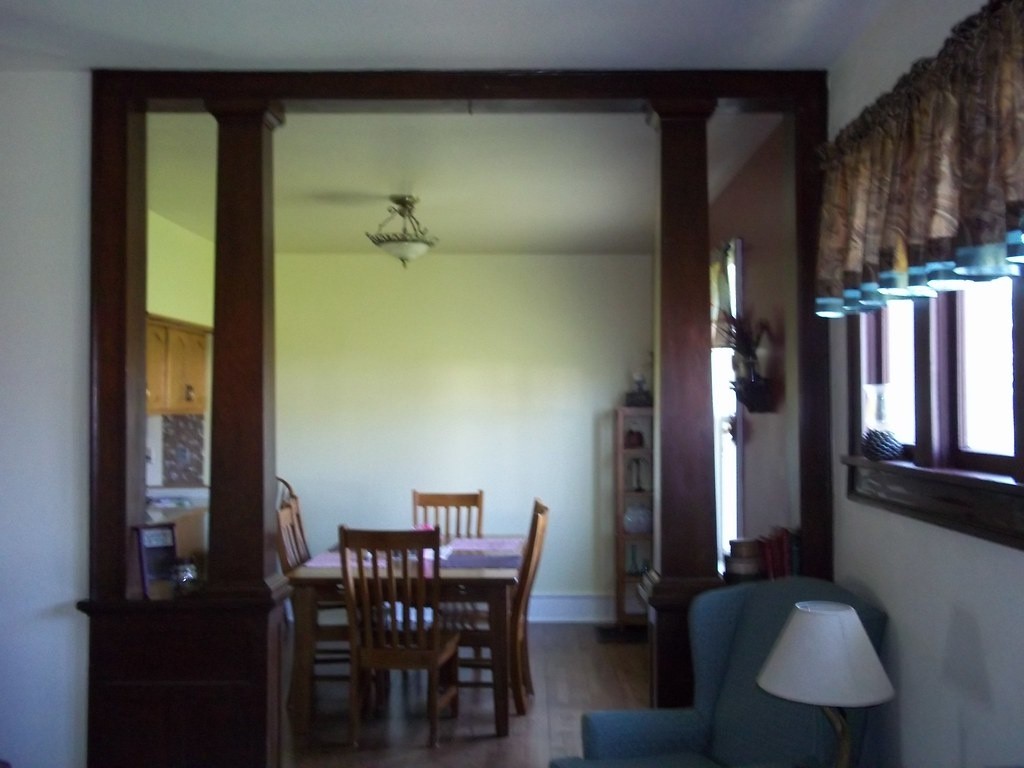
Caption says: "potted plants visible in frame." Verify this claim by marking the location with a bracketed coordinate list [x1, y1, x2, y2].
[720, 314, 779, 412]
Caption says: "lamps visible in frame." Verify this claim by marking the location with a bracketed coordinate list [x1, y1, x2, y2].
[366, 195, 438, 269]
[754, 600, 895, 768]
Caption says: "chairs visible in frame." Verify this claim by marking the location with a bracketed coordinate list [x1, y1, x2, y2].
[550, 579, 890, 768]
[276, 491, 547, 754]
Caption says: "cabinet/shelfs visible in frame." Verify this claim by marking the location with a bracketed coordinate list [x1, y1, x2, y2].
[615, 406, 652, 621]
[146, 323, 207, 415]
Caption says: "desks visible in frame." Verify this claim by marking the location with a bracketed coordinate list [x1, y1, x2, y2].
[144, 505, 208, 596]
[286, 538, 526, 737]
